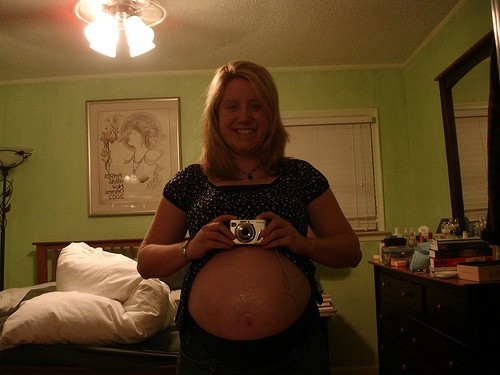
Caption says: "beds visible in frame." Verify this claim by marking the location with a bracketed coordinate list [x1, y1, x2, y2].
[0, 238, 189, 375]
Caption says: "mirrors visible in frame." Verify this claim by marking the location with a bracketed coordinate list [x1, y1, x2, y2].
[434, 29, 491, 236]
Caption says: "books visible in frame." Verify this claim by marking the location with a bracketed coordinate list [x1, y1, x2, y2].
[429, 238, 496, 267]
[316, 294, 337, 317]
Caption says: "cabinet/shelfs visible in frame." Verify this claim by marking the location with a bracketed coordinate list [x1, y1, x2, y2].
[368, 260, 500, 375]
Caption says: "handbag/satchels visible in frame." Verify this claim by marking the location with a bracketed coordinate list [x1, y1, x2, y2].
[409, 242, 432, 271]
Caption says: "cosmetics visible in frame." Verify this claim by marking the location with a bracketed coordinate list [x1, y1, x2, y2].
[394, 226, 417, 248]
[468, 216, 486, 236]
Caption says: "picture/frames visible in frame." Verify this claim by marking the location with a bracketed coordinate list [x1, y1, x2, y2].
[85, 96, 183, 217]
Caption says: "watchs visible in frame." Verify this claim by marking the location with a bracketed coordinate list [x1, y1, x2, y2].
[180, 241, 193, 260]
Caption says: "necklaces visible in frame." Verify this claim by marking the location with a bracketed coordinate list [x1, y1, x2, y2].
[237, 164, 261, 180]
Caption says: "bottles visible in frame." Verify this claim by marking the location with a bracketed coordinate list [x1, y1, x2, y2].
[480, 216, 486, 231]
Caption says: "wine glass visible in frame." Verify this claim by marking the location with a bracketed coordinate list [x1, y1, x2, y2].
[448, 218, 459, 240]
[441, 219, 451, 240]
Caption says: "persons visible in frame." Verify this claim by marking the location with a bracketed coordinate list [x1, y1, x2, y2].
[136, 61, 365, 375]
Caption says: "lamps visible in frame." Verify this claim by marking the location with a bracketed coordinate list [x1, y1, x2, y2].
[74, 0, 166, 58]
[0, 146, 35, 290]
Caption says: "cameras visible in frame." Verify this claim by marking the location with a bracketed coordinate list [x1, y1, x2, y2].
[229, 220, 266, 245]
